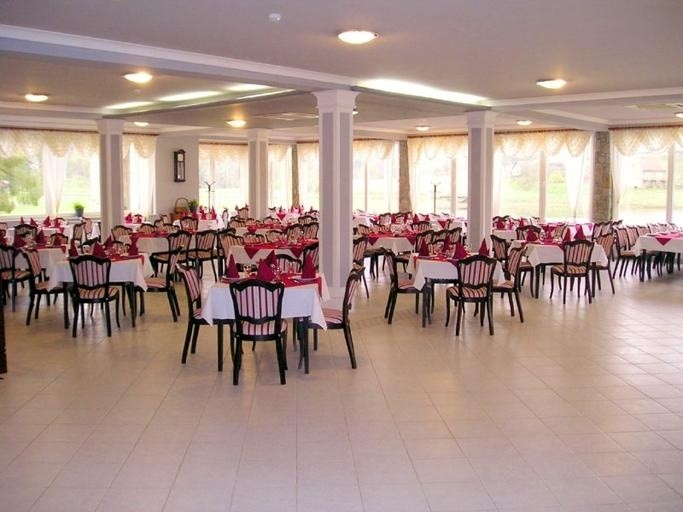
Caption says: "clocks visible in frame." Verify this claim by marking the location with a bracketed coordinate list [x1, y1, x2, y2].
[174, 149, 186, 181]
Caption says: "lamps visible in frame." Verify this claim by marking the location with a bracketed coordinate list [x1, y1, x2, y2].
[122, 71, 153, 84]
[25, 93, 48, 103]
[224, 120, 246, 127]
[336, 28, 379, 46]
[517, 120, 532, 127]
[134, 121, 149, 126]
[535, 77, 567, 90]
[416, 125, 429, 132]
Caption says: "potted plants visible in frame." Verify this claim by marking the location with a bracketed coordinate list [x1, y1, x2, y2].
[188, 200, 199, 213]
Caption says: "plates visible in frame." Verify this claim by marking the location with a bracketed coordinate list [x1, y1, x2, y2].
[221, 274, 240, 279]
[270, 279, 277, 284]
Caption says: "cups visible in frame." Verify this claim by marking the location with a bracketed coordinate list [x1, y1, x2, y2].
[437, 242, 443, 252]
[83, 244, 90, 254]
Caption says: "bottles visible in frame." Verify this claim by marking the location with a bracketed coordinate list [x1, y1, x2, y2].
[244, 265, 251, 277]
[291, 231, 295, 244]
[432, 244, 437, 255]
[449, 244, 453, 253]
[276, 268, 281, 280]
[428, 244, 432, 253]
